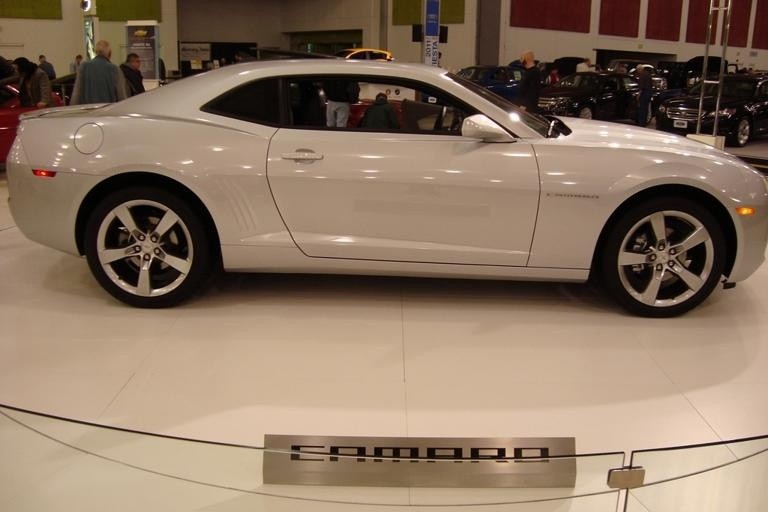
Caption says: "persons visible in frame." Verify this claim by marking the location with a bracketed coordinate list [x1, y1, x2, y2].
[357, 92, 401, 129]
[69, 40, 145, 106]
[636, 63, 652, 126]
[322, 51, 360, 128]
[513, 50, 541, 113]
[534, 63, 560, 88]
[0, 55, 56, 108]
[576, 57, 593, 73]
[219, 51, 245, 67]
